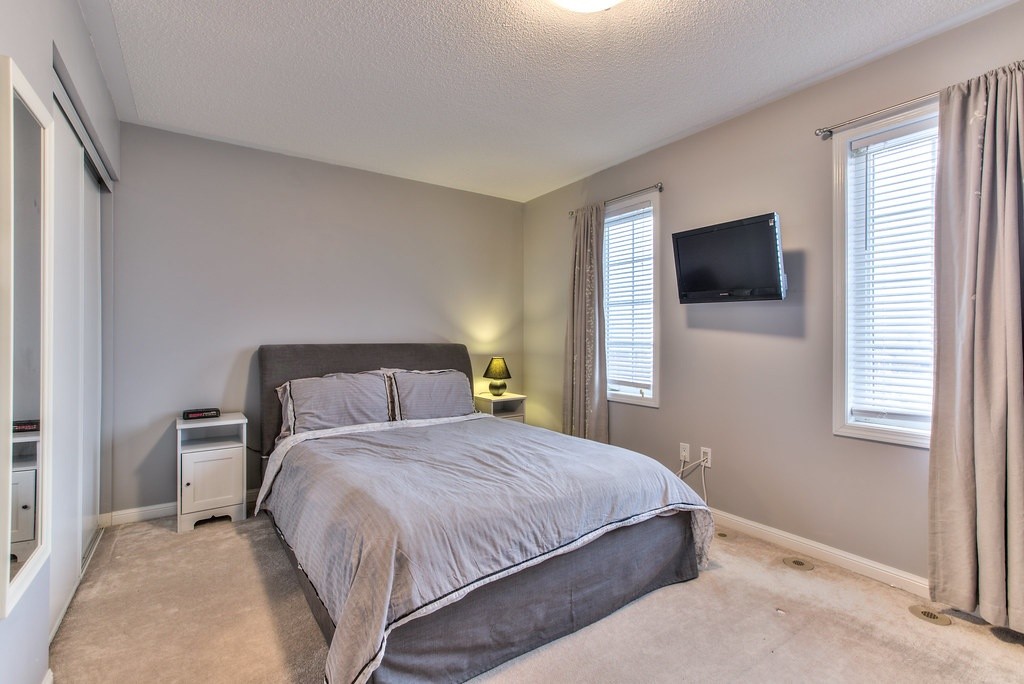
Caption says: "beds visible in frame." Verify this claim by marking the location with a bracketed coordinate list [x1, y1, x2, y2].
[255, 343, 716, 684]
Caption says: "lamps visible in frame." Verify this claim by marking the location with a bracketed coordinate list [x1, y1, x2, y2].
[482, 358, 511, 396]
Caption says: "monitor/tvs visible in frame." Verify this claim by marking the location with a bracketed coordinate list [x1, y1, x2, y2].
[671, 212, 787, 304]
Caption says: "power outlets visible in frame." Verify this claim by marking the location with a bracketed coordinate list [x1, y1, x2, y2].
[680, 443, 690, 462]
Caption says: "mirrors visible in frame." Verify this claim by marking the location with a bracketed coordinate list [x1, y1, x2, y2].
[0, 56, 53, 616]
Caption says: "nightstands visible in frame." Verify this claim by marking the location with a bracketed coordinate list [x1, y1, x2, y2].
[475, 392, 527, 423]
[176, 412, 248, 533]
[11, 431, 40, 551]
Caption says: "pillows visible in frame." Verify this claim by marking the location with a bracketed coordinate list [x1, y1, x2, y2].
[394, 372, 475, 420]
[292, 372, 393, 433]
